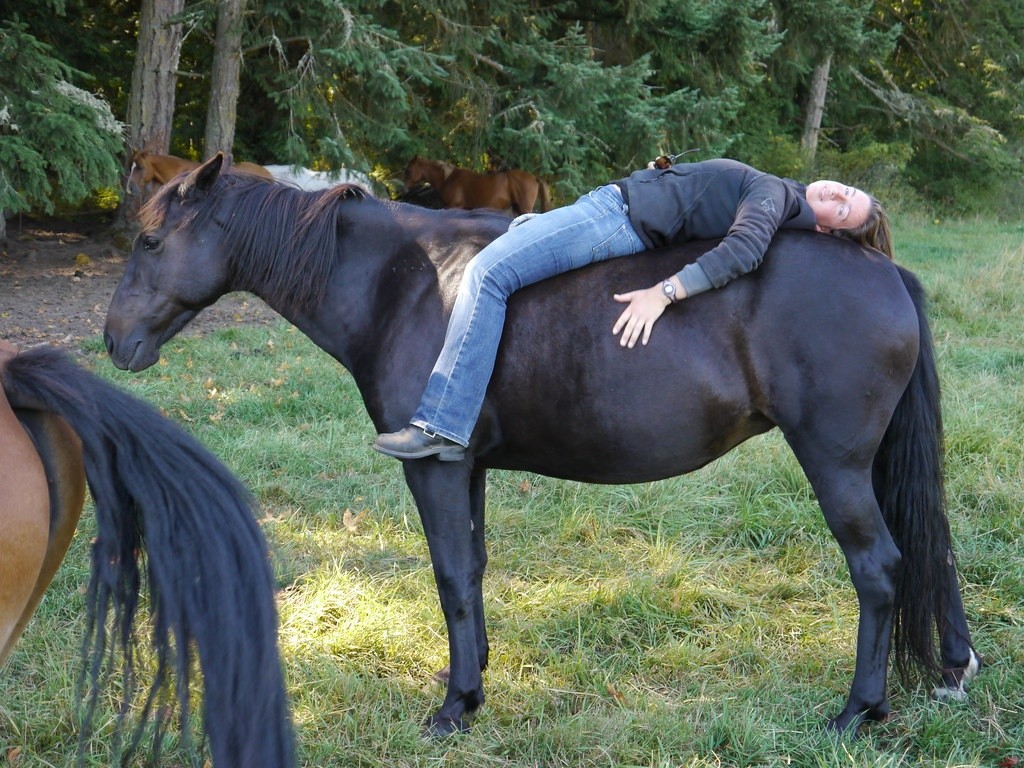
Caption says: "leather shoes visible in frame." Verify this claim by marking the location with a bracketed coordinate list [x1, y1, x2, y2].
[373, 423, 466, 461]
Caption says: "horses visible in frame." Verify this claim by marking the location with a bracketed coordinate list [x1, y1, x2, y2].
[0, 340, 303, 763]
[105, 150, 979, 738]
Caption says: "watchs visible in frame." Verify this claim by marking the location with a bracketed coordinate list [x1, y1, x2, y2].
[662, 279, 677, 303]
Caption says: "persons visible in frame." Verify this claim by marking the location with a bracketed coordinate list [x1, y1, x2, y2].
[371, 157, 894, 461]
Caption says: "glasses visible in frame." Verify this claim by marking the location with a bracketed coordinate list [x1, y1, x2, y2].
[654, 148, 701, 170]
